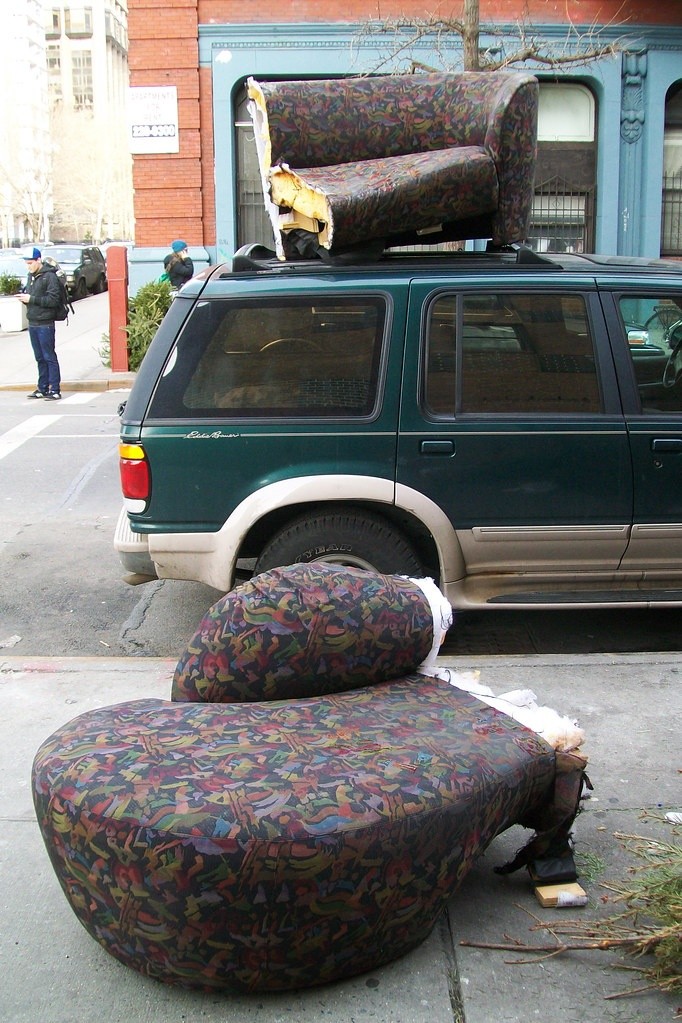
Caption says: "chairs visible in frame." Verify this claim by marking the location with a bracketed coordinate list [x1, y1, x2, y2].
[426, 309, 594, 408]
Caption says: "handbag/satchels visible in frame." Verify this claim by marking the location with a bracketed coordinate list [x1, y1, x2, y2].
[158, 271, 172, 284]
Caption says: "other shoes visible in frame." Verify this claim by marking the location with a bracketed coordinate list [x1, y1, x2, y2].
[44, 393, 62, 401]
[27, 388, 48, 398]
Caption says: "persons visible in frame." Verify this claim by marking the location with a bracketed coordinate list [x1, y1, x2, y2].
[154, 254, 173, 285]
[169, 241, 193, 289]
[13, 247, 61, 401]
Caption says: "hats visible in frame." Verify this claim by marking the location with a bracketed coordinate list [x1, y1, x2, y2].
[172, 241, 187, 253]
[20, 247, 41, 259]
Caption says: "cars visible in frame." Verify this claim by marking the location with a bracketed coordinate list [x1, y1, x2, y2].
[2, 239, 135, 305]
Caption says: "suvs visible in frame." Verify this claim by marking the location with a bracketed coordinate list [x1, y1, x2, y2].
[106, 240, 681, 629]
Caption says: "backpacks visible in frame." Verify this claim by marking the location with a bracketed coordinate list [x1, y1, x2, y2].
[54, 268, 74, 326]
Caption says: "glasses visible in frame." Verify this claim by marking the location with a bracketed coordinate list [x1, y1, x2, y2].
[184, 249, 188, 252]
